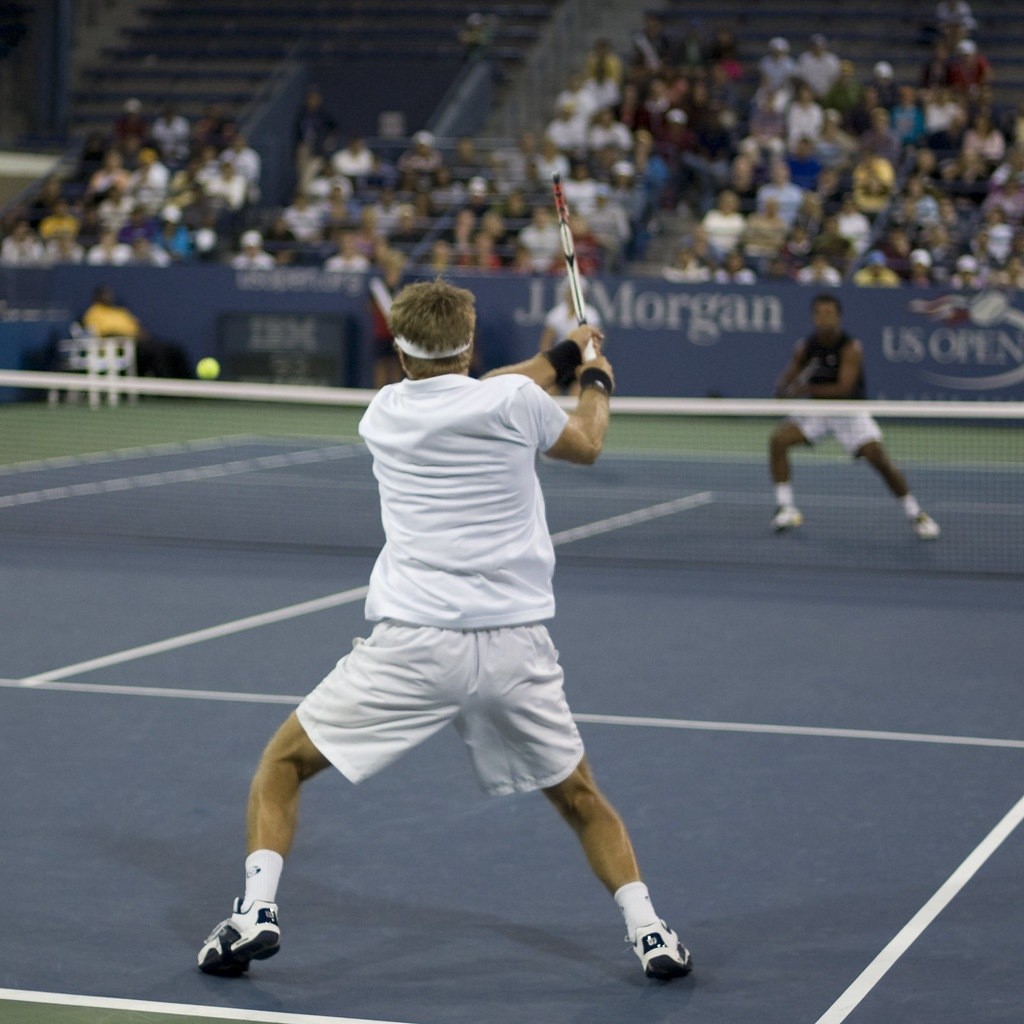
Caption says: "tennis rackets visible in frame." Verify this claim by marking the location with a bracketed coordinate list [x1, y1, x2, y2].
[553, 173, 596, 366]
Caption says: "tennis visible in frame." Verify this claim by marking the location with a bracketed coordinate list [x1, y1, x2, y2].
[196, 357, 220, 380]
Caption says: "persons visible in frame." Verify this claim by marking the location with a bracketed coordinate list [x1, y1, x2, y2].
[0, 0, 1024, 397]
[769, 294, 938, 541]
[200, 273, 695, 980]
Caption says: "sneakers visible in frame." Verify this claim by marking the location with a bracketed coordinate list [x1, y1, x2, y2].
[626, 919, 694, 981]
[911, 508, 942, 543]
[772, 503, 805, 532]
[197, 898, 282, 978]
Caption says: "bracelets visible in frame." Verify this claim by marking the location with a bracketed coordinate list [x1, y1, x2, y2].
[580, 367, 613, 396]
[542, 338, 581, 385]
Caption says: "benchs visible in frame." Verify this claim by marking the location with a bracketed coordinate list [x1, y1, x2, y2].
[59, 0, 1024, 280]
